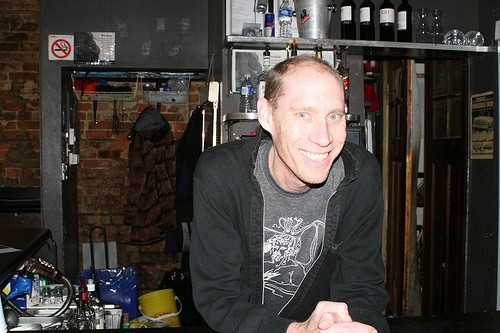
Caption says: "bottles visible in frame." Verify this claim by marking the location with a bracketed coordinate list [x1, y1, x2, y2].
[257, 40, 349, 114]
[380, 0, 395, 42]
[10, 274, 130, 329]
[396, 0, 412, 42]
[279, 0, 292, 38]
[340, 0, 356, 40]
[144, 77, 190, 92]
[240, 74, 254, 113]
[359, 0, 375, 41]
[156, 239, 205, 327]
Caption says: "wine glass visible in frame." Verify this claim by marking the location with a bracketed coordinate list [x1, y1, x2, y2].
[429, 8, 444, 44]
[442, 27, 485, 46]
[416, 8, 431, 43]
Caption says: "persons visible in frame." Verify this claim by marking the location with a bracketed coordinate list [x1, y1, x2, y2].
[189, 55, 392, 333]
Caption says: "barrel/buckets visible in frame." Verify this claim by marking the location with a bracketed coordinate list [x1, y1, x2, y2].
[291, 0, 336, 39]
[138, 288, 184, 327]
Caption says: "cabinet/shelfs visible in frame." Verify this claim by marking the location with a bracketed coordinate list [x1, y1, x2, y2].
[221, 35, 497, 122]
